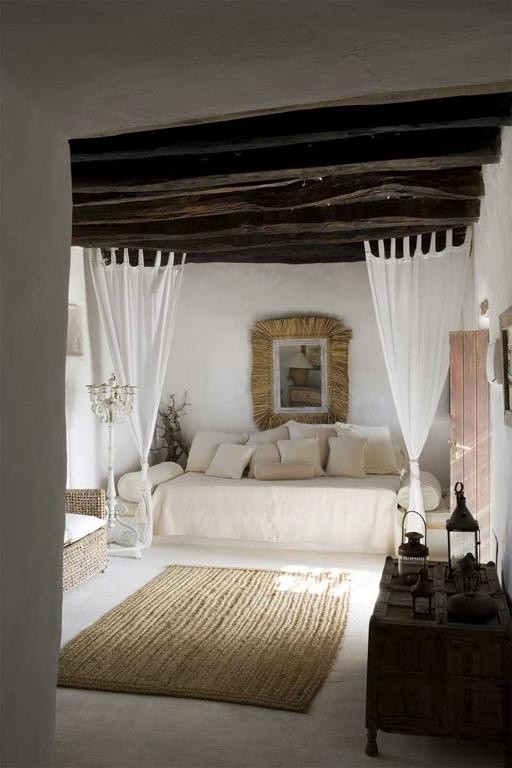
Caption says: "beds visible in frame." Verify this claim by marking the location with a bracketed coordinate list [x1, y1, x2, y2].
[116, 420, 442, 554]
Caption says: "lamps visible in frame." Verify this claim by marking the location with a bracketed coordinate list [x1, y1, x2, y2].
[87, 373, 138, 548]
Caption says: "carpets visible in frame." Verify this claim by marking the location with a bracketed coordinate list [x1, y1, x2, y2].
[57, 565, 352, 712]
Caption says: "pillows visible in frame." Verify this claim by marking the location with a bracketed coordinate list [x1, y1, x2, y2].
[277, 437, 325, 476]
[288, 420, 338, 468]
[64, 513, 108, 547]
[326, 437, 367, 478]
[335, 422, 400, 475]
[205, 444, 254, 479]
[249, 424, 289, 478]
[185, 432, 249, 471]
[254, 464, 313, 480]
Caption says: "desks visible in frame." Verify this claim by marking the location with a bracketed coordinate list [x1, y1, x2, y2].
[365, 556, 511, 757]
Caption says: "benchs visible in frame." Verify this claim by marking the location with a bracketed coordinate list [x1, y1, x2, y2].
[63, 489, 107, 592]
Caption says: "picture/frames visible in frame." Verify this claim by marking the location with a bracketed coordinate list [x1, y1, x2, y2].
[499, 305, 512, 429]
[273, 339, 328, 414]
